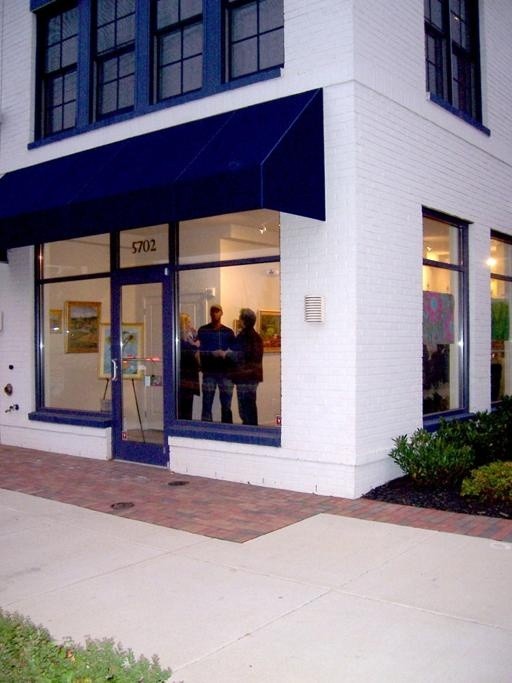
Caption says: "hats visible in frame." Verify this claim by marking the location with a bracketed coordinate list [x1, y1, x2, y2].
[210, 304, 222, 310]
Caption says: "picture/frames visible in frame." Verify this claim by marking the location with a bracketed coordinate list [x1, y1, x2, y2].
[256, 310, 281, 353]
[49, 309, 63, 334]
[99, 322, 145, 381]
[233, 319, 241, 336]
[63, 300, 101, 352]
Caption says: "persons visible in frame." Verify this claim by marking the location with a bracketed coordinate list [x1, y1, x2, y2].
[195, 304, 235, 423]
[178, 313, 200, 421]
[222, 309, 263, 425]
